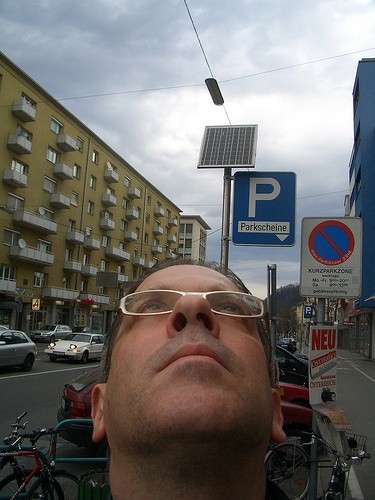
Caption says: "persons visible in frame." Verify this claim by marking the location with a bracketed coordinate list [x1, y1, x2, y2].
[91, 258, 286, 500]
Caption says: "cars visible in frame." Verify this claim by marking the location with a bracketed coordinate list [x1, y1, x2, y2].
[54, 365, 321, 461]
[43, 332, 106, 364]
[0, 330, 37, 371]
[30, 324, 91, 343]
[273, 338, 312, 409]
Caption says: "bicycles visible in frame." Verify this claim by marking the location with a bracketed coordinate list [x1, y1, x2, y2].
[0, 411, 79, 500]
[264, 430, 371, 500]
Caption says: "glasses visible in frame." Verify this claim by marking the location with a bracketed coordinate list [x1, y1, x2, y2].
[104, 291, 273, 368]
